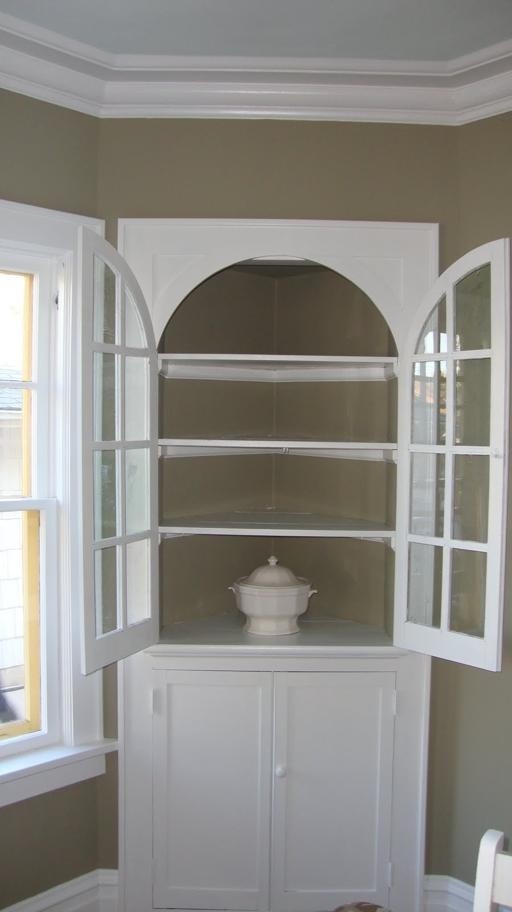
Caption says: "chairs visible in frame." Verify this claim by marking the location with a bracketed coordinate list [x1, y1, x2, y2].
[471, 829, 510, 911]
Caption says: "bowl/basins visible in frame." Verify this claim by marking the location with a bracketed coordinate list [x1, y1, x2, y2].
[228, 554, 318, 636]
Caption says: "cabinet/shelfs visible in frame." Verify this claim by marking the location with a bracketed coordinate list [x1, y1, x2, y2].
[115, 218, 439, 912]
